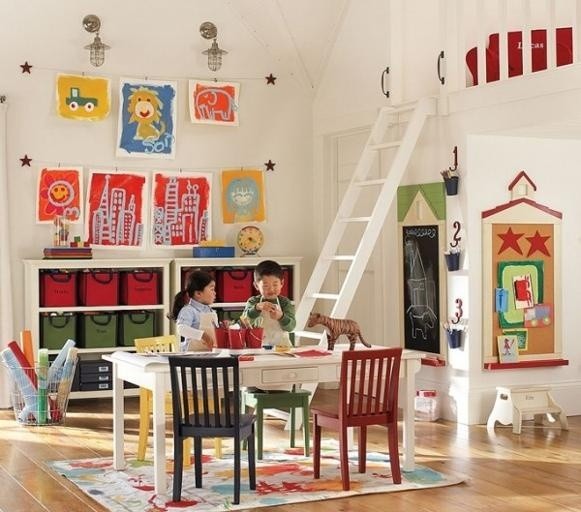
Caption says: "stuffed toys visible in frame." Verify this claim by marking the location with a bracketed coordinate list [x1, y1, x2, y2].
[306, 312, 371, 351]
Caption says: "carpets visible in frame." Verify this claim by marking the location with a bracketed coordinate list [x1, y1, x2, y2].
[43, 437, 466, 512]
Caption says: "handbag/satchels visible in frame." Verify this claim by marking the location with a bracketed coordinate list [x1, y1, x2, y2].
[184, 270, 288, 302]
[41, 270, 158, 307]
[41, 312, 155, 349]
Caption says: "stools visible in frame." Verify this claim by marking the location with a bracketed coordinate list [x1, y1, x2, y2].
[486, 384, 569, 435]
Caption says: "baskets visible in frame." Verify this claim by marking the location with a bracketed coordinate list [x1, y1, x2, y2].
[2, 361, 76, 425]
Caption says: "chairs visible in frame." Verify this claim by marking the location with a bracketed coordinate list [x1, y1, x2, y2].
[134, 335, 221, 469]
[309, 346, 403, 491]
[167, 354, 259, 505]
[243, 333, 312, 460]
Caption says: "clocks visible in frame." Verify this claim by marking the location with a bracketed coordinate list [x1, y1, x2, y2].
[236, 226, 265, 258]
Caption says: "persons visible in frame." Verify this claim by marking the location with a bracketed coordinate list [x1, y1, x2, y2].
[165, 270, 221, 351]
[242, 259, 297, 394]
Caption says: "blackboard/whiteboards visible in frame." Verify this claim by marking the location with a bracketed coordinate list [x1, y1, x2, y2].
[398, 220, 448, 356]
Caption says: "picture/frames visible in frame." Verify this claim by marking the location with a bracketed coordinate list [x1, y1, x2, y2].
[497, 335, 520, 363]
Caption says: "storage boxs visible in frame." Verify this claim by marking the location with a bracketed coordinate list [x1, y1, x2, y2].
[80, 362, 113, 390]
[123, 271, 159, 305]
[119, 311, 156, 347]
[220, 311, 243, 321]
[81, 312, 118, 348]
[182, 267, 289, 302]
[40, 272, 77, 307]
[81, 270, 119, 306]
[42, 313, 76, 349]
[49, 363, 80, 391]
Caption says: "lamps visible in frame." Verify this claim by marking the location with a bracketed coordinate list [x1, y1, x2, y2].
[82, 14, 112, 68]
[199, 21, 228, 71]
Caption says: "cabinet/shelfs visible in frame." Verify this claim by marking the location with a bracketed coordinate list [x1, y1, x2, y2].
[22, 259, 171, 399]
[170, 257, 300, 320]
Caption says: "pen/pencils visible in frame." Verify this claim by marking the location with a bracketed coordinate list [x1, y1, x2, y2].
[239, 315, 263, 330]
[211, 319, 225, 329]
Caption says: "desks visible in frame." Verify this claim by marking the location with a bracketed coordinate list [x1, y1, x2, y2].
[102, 343, 427, 496]
